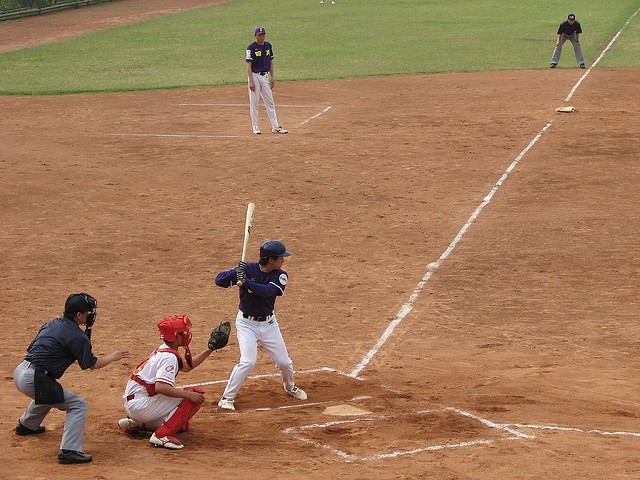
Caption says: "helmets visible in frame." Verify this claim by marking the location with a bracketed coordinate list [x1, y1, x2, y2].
[157, 316, 192, 345]
[64, 293, 97, 327]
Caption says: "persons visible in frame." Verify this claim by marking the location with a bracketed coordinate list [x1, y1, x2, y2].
[245, 28, 289, 134]
[15, 292, 131, 466]
[550, 13, 587, 68]
[214, 238, 309, 411]
[118, 316, 230, 451]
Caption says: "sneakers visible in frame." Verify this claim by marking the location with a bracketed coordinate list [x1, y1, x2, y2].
[15, 423, 46, 434]
[150, 432, 184, 450]
[284, 385, 309, 401]
[59, 449, 92, 463]
[218, 397, 235, 411]
[272, 128, 288, 134]
[119, 419, 138, 433]
[253, 130, 260, 134]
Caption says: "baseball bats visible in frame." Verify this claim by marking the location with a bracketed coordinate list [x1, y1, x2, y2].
[236, 202, 255, 287]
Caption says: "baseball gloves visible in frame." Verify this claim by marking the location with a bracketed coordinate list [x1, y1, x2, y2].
[207, 321, 231, 351]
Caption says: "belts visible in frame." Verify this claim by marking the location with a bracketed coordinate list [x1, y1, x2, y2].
[28, 364, 37, 371]
[256, 72, 269, 75]
[242, 310, 275, 321]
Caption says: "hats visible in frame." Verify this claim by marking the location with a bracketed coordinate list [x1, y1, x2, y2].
[255, 27, 266, 35]
[569, 13, 575, 20]
[264, 241, 294, 259]
